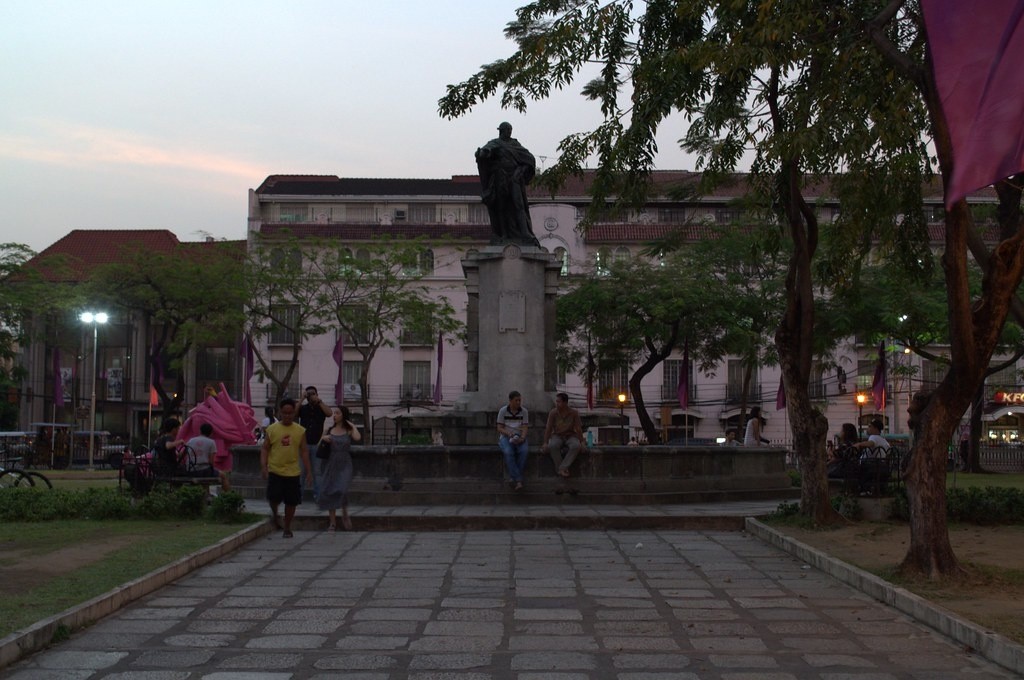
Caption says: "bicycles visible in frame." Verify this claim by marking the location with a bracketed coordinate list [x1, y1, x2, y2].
[0, 457, 52, 488]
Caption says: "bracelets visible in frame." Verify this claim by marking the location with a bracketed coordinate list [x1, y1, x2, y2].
[520, 436, 526, 442]
[317, 399, 323, 404]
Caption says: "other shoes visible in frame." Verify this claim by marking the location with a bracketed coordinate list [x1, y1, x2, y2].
[327, 523, 336, 535]
[283, 530, 292, 538]
[274, 516, 282, 530]
[557, 467, 570, 478]
[508, 478, 521, 490]
[342, 518, 353, 532]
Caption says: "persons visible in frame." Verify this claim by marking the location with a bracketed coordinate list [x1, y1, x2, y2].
[37, 425, 136, 464]
[827, 418, 890, 477]
[187, 423, 231, 492]
[542, 393, 587, 476]
[827, 439, 837, 461]
[294, 386, 333, 504]
[155, 419, 187, 479]
[316, 406, 361, 531]
[475, 123, 536, 246]
[262, 406, 278, 434]
[628, 436, 638, 446]
[719, 406, 770, 446]
[496, 391, 529, 489]
[261, 398, 314, 539]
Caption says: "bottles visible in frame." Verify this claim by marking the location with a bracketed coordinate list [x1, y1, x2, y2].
[588, 431, 593, 448]
[514, 432, 520, 440]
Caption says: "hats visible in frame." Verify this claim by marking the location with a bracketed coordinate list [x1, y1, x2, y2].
[871, 417, 883, 433]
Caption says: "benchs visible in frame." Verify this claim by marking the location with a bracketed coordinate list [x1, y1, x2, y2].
[829, 445, 905, 500]
[133, 444, 223, 499]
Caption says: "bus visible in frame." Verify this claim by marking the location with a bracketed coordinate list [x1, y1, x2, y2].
[833, 434, 954, 472]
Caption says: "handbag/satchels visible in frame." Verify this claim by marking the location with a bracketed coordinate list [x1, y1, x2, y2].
[316, 427, 332, 459]
[559, 429, 577, 441]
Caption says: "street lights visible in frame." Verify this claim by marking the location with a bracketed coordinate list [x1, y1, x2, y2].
[858, 396, 864, 442]
[905, 348, 912, 406]
[618, 395, 626, 444]
[80, 311, 108, 470]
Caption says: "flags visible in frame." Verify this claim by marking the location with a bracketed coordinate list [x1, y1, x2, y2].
[241, 331, 254, 404]
[870, 341, 889, 410]
[150, 334, 163, 406]
[586, 350, 597, 410]
[919, 0, 1023, 211]
[51, 342, 64, 405]
[678, 339, 688, 410]
[776, 374, 788, 410]
[332, 333, 342, 404]
